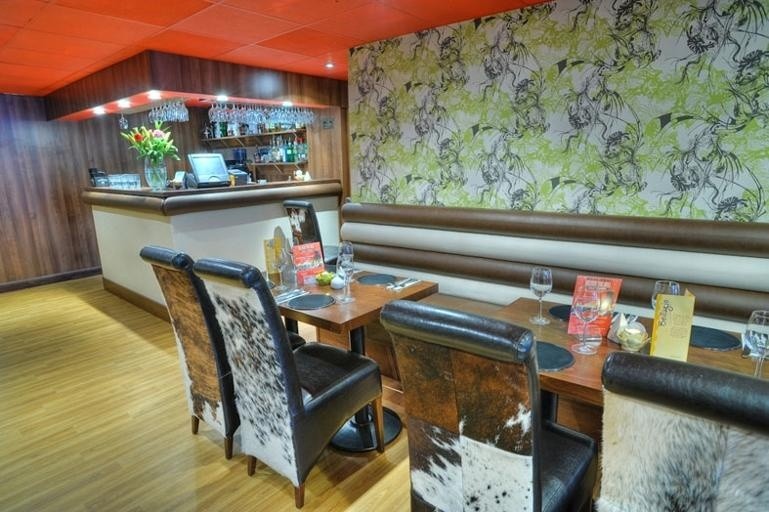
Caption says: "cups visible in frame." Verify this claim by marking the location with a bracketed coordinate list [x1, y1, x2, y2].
[108, 173, 142, 189]
[651, 278, 680, 306]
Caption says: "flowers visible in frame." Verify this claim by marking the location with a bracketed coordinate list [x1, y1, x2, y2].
[119, 120, 182, 185]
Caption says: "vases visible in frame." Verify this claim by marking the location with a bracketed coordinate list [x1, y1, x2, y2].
[144, 158, 169, 191]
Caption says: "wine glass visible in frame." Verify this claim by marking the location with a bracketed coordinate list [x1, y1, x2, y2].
[147, 100, 190, 124]
[336, 241, 355, 303]
[117, 111, 129, 130]
[569, 276, 602, 356]
[204, 101, 317, 124]
[746, 308, 769, 378]
[528, 264, 550, 327]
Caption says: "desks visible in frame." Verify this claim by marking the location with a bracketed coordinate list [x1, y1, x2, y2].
[270, 267, 437, 453]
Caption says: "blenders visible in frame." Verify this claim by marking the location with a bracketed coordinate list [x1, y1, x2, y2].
[232, 148, 248, 165]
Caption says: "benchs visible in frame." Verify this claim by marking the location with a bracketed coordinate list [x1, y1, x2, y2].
[340, 203, 769, 337]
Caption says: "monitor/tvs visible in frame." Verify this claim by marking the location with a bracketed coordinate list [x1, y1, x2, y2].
[187, 153, 231, 187]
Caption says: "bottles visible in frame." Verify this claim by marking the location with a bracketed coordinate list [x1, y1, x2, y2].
[198, 121, 307, 138]
[250, 135, 308, 163]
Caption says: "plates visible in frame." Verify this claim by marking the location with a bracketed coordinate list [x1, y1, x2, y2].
[357, 271, 395, 288]
[681, 324, 743, 353]
[522, 341, 575, 374]
[287, 294, 335, 310]
[545, 303, 574, 323]
